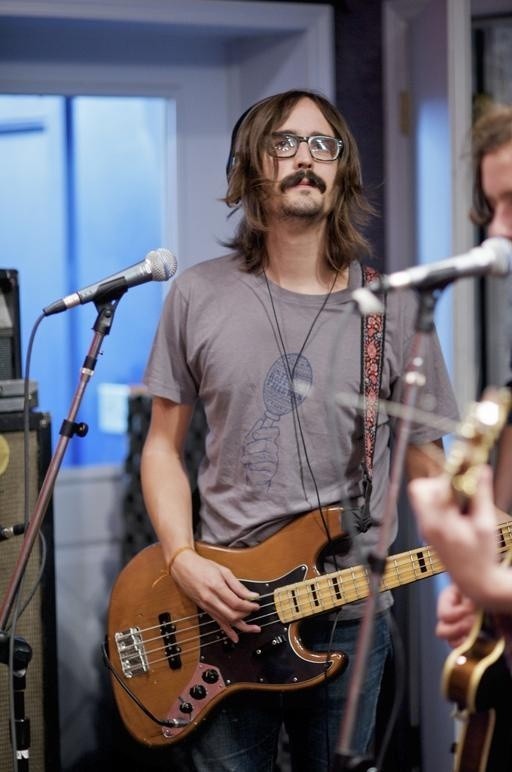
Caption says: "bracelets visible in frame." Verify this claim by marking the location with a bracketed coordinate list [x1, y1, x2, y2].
[168, 545, 193, 576]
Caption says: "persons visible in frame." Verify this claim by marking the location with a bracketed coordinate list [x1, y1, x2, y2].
[406, 98, 512, 772]
[138, 85, 463, 772]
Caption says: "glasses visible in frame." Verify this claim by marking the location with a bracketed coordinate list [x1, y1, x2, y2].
[265, 131, 347, 164]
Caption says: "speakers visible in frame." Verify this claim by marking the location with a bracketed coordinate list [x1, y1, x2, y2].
[0, 409, 62, 772]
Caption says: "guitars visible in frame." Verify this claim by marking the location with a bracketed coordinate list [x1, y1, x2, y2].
[101, 506, 511, 745]
[439, 388, 510, 771]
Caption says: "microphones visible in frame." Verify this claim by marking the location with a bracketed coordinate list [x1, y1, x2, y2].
[368, 236, 512, 298]
[43, 248, 177, 316]
